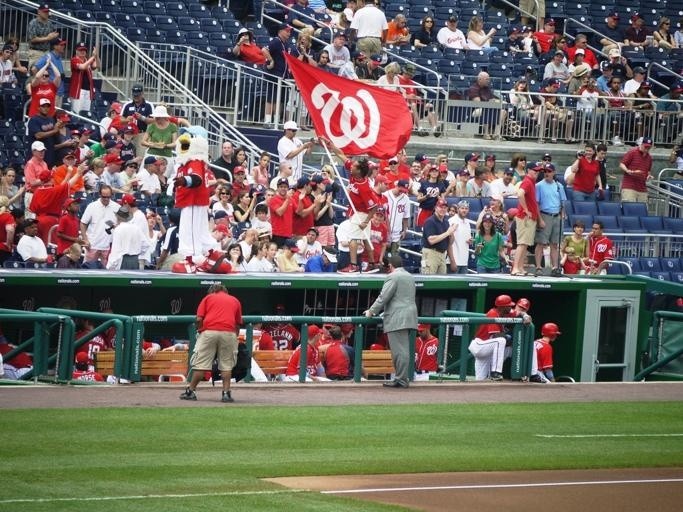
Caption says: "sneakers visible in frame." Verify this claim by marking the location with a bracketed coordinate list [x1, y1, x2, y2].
[337, 264, 360, 274]
[222, 391, 233, 402]
[535, 269, 543, 277]
[550, 269, 561, 277]
[361, 264, 380, 274]
[179, 387, 197, 401]
[487, 371, 503, 381]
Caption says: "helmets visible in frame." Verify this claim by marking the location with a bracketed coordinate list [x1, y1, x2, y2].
[518, 298, 530, 311]
[495, 295, 515, 307]
[417, 323, 432, 331]
[541, 323, 561, 339]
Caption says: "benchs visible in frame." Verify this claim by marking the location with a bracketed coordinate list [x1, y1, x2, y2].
[93, 343, 399, 381]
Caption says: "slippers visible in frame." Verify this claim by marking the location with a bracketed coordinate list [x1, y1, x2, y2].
[511, 271, 523, 276]
[524, 271, 533, 276]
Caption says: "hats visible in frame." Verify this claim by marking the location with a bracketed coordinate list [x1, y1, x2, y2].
[402, 63, 416, 77]
[309, 323, 354, 338]
[278, 23, 293, 32]
[214, 141, 330, 237]
[149, 106, 171, 119]
[507, 10, 682, 94]
[283, 121, 298, 131]
[334, 31, 381, 65]
[50, 38, 66, 45]
[75, 353, 96, 365]
[642, 136, 652, 148]
[39, 4, 50, 13]
[75, 41, 87, 51]
[39, 98, 51, 107]
[235, 28, 253, 41]
[274, 304, 285, 312]
[132, 85, 143, 95]
[23, 124, 209, 228]
[355, 148, 555, 221]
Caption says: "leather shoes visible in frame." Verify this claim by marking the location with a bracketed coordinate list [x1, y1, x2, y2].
[383, 381, 400, 387]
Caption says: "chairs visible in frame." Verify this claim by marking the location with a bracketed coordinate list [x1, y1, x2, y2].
[287, 159, 681, 285]
[0, 78, 175, 271]
[35, 0, 682, 138]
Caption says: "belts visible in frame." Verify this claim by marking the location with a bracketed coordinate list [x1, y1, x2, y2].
[424, 246, 446, 254]
